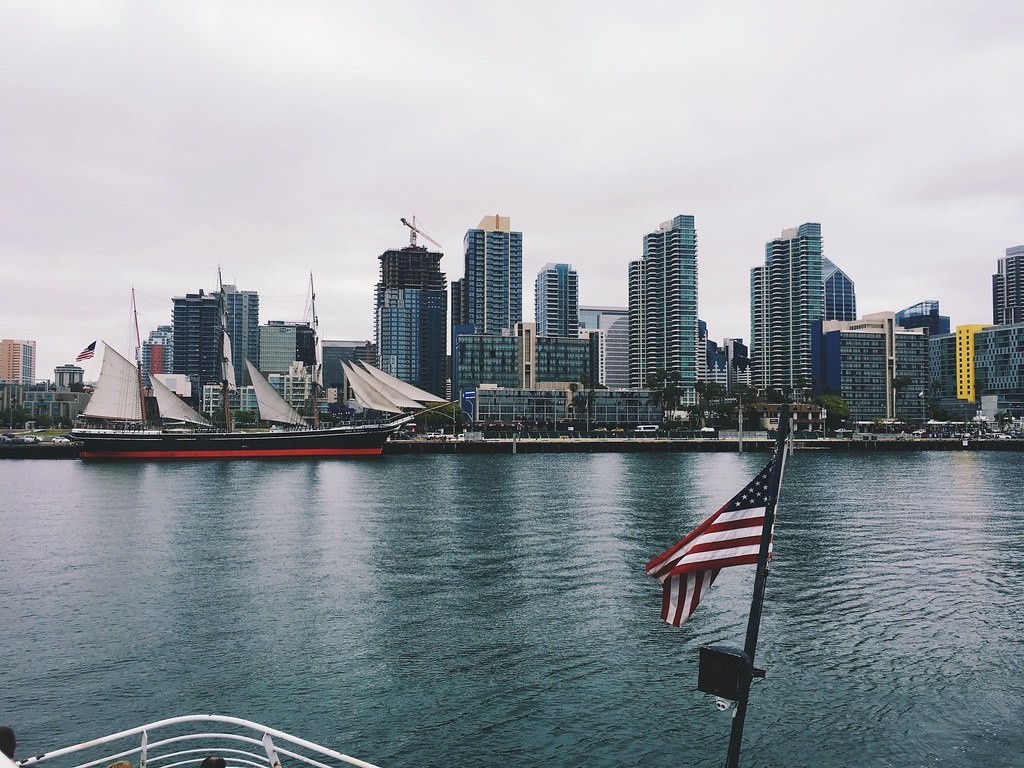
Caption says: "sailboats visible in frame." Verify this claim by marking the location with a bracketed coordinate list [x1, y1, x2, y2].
[69, 263, 459, 461]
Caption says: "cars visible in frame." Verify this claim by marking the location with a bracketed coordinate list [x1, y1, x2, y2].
[611, 428, 624, 431]
[24, 435, 42, 443]
[594, 426, 607, 432]
[52, 436, 70, 443]
[992, 433, 1011, 439]
[0, 436, 12, 443]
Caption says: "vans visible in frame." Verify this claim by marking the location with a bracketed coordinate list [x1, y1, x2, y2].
[425, 432, 443, 440]
[634, 425, 659, 431]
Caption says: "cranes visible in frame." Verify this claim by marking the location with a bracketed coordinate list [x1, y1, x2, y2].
[400, 214, 442, 250]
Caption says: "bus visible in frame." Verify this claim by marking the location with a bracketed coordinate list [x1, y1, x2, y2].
[853, 420, 984, 438]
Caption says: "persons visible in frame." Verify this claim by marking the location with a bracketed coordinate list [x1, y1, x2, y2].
[517, 421, 525, 441]
[434, 432, 459, 443]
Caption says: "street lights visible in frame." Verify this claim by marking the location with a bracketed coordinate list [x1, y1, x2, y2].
[960, 405, 967, 433]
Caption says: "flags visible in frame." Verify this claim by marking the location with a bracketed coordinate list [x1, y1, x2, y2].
[646, 449, 786, 627]
[919, 390, 924, 397]
[76, 340, 96, 363]
